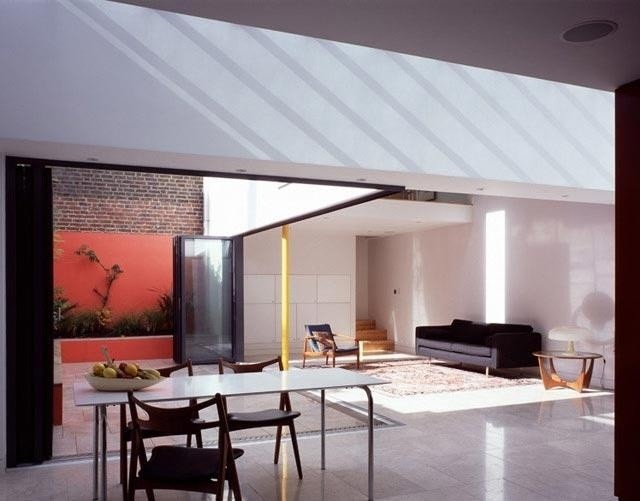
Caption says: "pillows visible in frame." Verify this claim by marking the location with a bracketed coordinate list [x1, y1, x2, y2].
[312, 331, 337, 352]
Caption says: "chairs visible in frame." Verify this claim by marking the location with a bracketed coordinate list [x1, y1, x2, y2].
[122, 389, 242, 501]
[302, 324, 359, 370]
[218, 356, 303, 479]
[119, 359, 203, 486]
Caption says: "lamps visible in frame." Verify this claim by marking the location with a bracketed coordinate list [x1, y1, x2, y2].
[548, 326, 581, 356]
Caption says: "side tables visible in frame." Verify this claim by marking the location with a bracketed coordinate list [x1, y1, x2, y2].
[532, 350, 603, 393]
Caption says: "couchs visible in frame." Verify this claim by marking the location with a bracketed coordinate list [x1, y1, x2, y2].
[415, 319, 541, 379]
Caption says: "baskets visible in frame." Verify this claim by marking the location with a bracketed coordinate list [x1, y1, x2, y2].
[84, 367, 166, 391]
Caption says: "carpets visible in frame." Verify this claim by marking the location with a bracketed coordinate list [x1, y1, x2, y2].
[294, 357, 541, 399]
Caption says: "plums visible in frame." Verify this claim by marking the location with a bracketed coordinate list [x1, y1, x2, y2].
[93, 360, 141, 379]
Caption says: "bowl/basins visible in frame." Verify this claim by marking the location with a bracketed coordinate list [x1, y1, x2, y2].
[85, 374, 163, 392]
